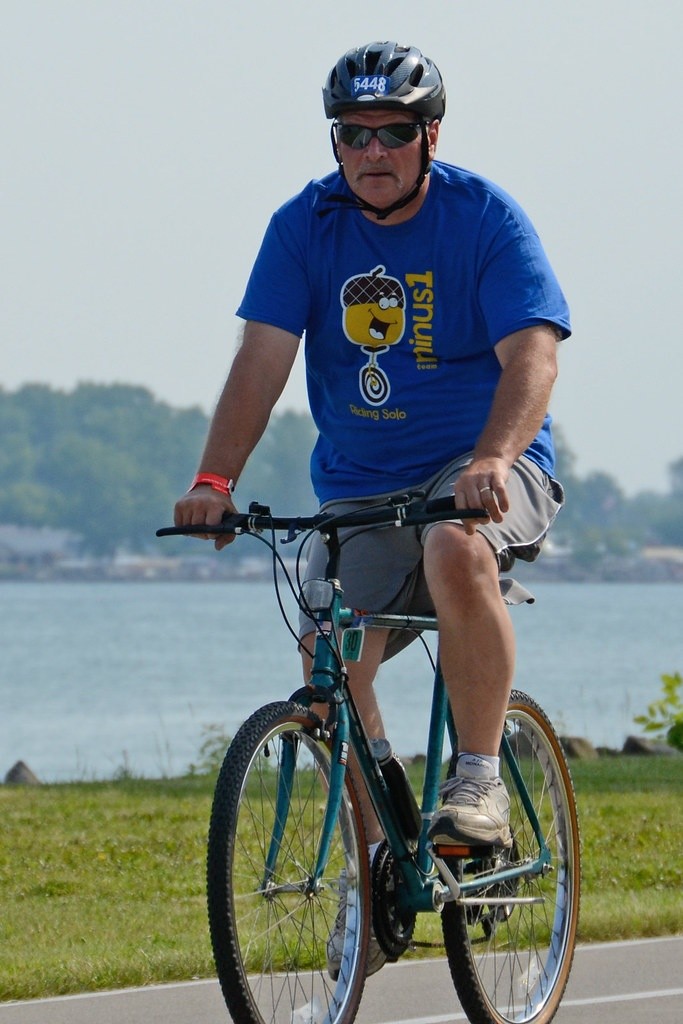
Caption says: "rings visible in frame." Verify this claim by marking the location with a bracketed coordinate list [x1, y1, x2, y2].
[480, 486, 490, 493]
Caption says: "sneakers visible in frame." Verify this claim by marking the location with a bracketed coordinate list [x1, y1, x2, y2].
[427, 753, 514, 850]
[326, 839, 404, 981]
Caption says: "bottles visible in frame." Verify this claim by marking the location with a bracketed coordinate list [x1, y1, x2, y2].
[371, 738, 422, 840]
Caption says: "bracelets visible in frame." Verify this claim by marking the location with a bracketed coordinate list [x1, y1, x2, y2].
[187, 471, 236, 495]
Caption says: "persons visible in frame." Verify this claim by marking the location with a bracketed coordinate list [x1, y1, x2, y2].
[173, 41, 571, 980]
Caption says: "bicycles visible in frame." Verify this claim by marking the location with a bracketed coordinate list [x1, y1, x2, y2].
[154, 489, 583, 1023]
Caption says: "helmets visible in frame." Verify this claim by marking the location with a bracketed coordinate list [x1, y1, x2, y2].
[322, 41, 446, 125]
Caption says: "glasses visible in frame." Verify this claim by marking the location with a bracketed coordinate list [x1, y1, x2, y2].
[332, 118, 420, 149]
